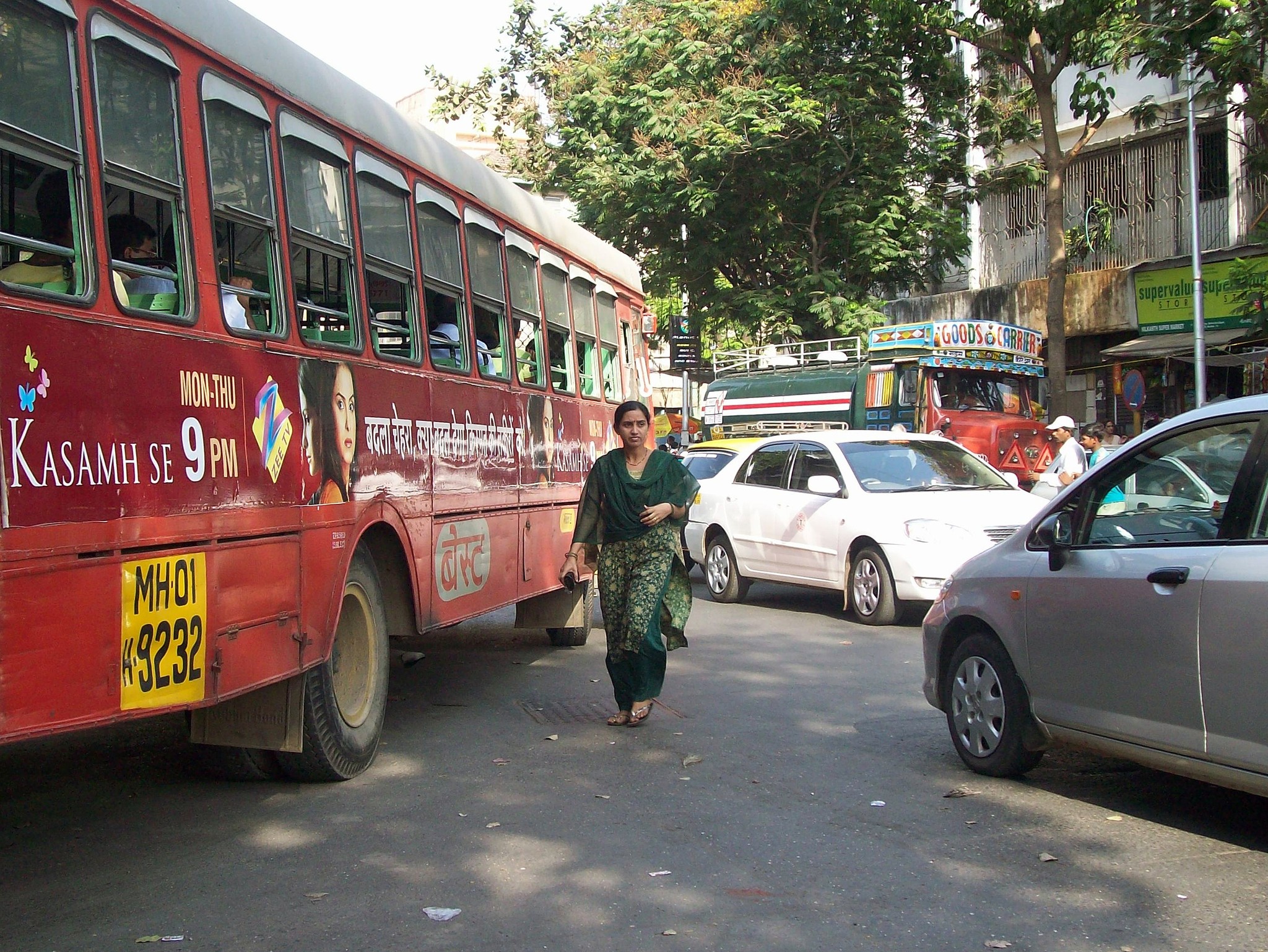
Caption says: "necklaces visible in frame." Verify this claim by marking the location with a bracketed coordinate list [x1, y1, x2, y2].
[627, 447, 648, 466]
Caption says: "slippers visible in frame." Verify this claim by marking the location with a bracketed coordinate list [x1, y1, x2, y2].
[608, 714, 630, 726]
[628, 703, 654, 726]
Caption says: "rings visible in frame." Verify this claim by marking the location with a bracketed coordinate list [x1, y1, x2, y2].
[655, 518, 657, 521]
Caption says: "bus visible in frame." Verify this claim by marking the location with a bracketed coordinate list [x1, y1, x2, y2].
[0, 0, 646, 782]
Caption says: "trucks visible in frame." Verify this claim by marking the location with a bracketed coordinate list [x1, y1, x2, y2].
[702, 319, 1059, 487]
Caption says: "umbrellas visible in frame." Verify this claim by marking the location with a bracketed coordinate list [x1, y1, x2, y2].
[654, 412, 703, 449]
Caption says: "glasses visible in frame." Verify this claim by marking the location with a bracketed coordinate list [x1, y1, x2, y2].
[132, 246, 157, 258]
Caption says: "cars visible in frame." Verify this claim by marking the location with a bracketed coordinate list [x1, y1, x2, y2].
[680, 437, 767, 481]
[683, 429, 1052, 626]
[922, 392, 1268, 798]
[1031, 446, 1242, 513]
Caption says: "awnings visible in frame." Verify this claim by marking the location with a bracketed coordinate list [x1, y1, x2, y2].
[1097, 328, 1249, 361]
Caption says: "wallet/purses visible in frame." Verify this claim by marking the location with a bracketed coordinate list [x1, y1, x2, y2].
[563, 571, 580, 591]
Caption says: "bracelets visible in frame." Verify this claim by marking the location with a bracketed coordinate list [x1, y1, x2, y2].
[667, 501, 674, 514]
[564, 553, 578, 561]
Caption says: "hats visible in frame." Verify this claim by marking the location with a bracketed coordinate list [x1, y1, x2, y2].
[1045, 415, 1078, 431]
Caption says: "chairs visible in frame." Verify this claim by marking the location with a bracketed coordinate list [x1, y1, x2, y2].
[884, 456, 912, 481]
[805, 456, 838, 480]
[0, 237, 567, 397]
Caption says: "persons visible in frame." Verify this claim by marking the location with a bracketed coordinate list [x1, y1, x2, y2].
[295, 357, 359, 506]
[0, 167, 540, 384]
[559, 401, 706, 728]
[525, 394, 560, 488]
[886, 415, 1189, 514]
